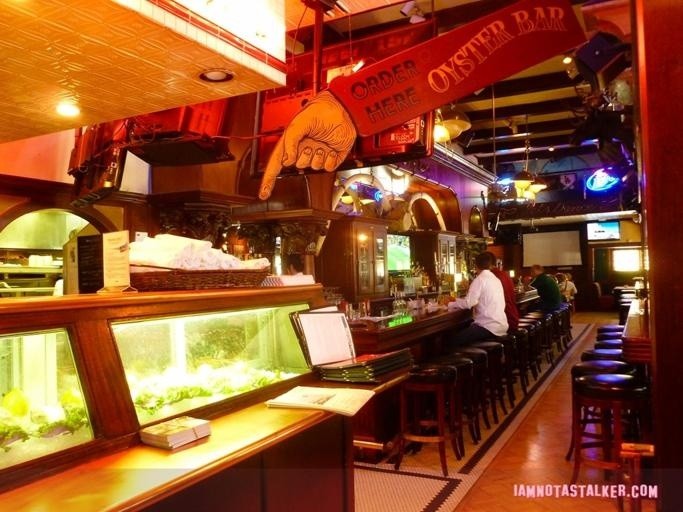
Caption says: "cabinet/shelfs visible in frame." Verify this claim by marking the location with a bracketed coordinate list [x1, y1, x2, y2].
[409, 226, 459, 284]
[319, 213, 395, 305]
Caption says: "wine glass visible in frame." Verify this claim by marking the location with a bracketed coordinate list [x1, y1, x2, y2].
[391, 291, 406, 313]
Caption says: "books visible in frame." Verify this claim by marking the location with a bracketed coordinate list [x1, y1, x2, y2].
[263, 347, 413, 417]
[140, 415, 213, 449]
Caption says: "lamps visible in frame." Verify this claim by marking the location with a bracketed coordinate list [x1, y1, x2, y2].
[440, 102, 473, 140]
[510, 114, 548, 195]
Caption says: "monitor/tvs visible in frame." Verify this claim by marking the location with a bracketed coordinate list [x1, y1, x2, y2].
[584, 220, 621, 241]
[387, 233, 415, 272]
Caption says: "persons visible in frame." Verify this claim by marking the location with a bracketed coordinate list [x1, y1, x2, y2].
[485, 252, 521, 376]
[442, 254, 510, 384]
[523, 263, 578, 341]
[258, 90, 356, 202]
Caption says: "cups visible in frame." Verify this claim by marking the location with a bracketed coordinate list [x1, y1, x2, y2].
[351, 310, 361, 322]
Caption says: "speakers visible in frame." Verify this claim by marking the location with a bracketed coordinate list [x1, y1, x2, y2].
[496, 229, 513, 243]
[573, 31, 632, 91]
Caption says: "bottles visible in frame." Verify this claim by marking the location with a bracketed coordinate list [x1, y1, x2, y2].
[272, 236, 284, 277]
[410, 257, 430, 286]
[414, 298, 425, 315]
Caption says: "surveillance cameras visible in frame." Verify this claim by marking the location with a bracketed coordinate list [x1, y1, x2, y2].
[400, 0, 415, 16]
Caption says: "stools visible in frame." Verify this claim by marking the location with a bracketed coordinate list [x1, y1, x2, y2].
[394, 302, 572, 480]
[564, 324, 649, 510]
[612, 284, 637, 324]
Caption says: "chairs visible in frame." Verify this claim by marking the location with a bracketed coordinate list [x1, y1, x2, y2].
[593, 282, 614, 308]
